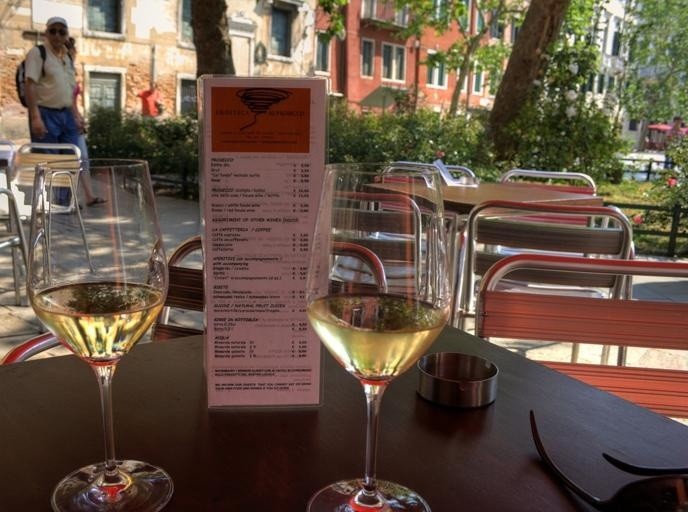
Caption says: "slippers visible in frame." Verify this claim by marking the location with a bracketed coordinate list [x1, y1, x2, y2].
[86, 197, 108, 207]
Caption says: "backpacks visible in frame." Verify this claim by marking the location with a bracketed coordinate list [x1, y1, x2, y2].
[15, 44, 47, 107]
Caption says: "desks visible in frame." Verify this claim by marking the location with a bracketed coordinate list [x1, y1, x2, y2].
[2, 323, 685, 512]
[366, 176, 604, 326]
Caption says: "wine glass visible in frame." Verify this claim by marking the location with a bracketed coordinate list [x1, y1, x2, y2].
[28, 158, 176, 512]
[305, 165, 449, 512]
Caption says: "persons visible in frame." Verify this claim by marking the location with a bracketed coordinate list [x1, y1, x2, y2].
[24, 17, 109, 215]
[129, 80, 165, 126]
[664, 117, 684, 170]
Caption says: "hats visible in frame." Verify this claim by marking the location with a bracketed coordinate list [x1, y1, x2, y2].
[46, 17, 68, 28]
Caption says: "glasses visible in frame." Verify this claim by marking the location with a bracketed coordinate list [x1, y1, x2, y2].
[48, 28, 66, 35]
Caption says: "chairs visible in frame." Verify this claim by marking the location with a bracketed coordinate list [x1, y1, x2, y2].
[500, 168, 596, 198]
[12, 141, 96, 284]
[329, 241, 390, 301]
[456, 200, 636, 368]
[330, 189, 423, 312]
[379, 161, 482, 190]
[474, 251, 687, 425]
